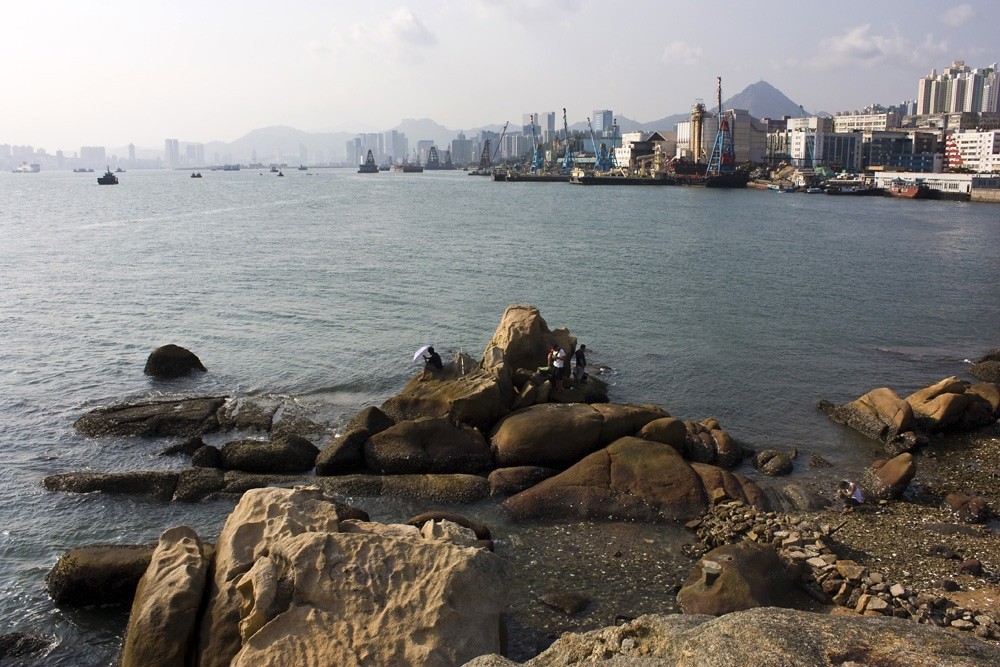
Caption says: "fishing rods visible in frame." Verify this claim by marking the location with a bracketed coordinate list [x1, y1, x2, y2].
[457, 311, 465, 374]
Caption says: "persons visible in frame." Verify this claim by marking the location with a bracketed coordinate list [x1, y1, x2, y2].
[419, 347, 443, 382]
[575, 344, 587, 390]
[837, 480, 863, 515]
[547, 342, 566, 390]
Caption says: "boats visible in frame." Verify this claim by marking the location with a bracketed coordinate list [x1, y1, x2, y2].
[97, 165, 119, 185]
[378, 153, 424, 174]
[12, 160, 40, 174]
[357, 149, 380, 173]
[190, 171, 203, 178]
[765, 167, 930, 199]
[463, 166, 509, 182]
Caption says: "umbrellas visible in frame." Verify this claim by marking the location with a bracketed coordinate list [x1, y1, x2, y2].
[413, 345, 433, 362]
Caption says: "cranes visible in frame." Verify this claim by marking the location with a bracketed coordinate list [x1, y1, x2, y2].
[799, 104, 818, 171]
[703, 73, 738, 180]
[526, 114, 545, 177]
[585, 116, 619, 173]
[557, 107, 575, 176]
